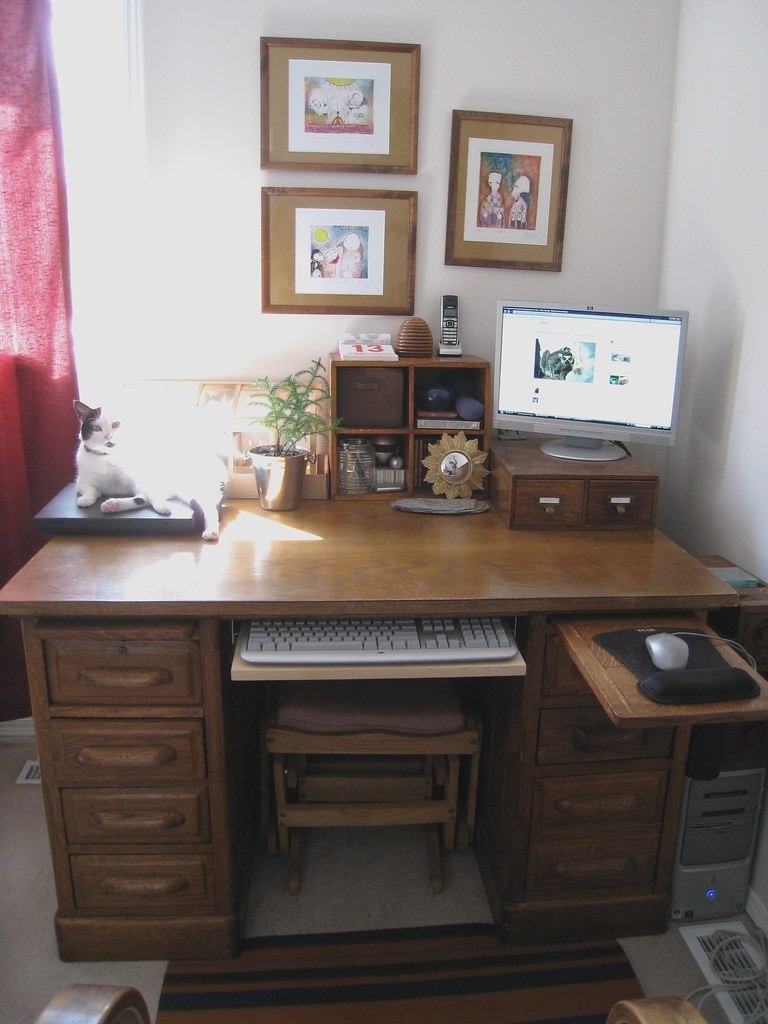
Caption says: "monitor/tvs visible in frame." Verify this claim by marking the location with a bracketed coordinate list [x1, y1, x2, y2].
[492, 300, 690, 462]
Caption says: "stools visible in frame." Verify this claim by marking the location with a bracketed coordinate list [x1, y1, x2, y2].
[263, 679, 484, 895]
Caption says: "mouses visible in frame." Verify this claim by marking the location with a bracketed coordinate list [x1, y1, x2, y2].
[646, 633, 690, 671]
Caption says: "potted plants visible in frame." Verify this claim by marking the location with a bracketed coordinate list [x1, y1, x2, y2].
[248, 357, 344, 511]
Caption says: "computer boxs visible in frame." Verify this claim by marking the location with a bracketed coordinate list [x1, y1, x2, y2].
[666, 733, 768, 925]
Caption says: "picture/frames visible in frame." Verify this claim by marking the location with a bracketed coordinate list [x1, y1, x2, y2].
[259, 37, 421, 174]
[260, 187, 418, 315]
[444, 109, 573, 273]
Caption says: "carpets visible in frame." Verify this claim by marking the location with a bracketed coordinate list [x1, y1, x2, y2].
[155, 936, 645, 1024]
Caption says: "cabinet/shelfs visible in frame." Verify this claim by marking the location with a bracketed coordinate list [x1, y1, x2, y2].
[489, 437, 659, 532]
[328, 353, 491, 501]
[0, 498, 768, 975]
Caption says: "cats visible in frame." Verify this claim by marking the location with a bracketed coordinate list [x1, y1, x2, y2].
[73, 400, 229, 541]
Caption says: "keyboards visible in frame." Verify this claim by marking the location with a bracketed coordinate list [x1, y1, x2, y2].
[240, 618, 519, 665]
